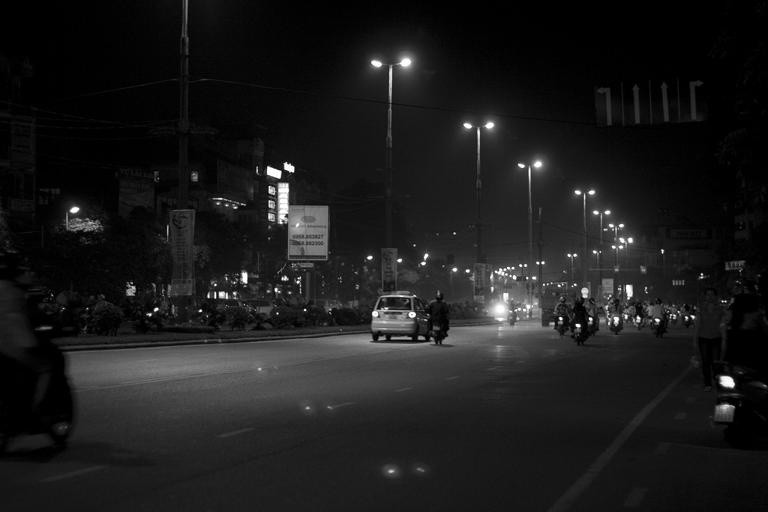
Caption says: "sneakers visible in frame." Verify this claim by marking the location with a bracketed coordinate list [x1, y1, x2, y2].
[704, 386, 712, 392]
[440, 331, 447, 336]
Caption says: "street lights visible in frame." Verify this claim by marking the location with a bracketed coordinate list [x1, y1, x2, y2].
[464, 122, 495, 199]
[593, 209, 634, 268]
[574, 189, 596, 280]
[371, 57, 411, 249]
[568, 254, 577, 280]
[518, 161, 542, 305]
[65, 203, 80, 231]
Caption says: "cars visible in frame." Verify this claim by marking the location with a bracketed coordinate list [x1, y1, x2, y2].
[370, 295, 430, 342]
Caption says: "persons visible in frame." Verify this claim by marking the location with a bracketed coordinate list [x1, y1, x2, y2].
[426, 291, 450, 337]
[553, 294, 696, 334]
[696, 274, 768, 392]
[0, 251, 57, 434]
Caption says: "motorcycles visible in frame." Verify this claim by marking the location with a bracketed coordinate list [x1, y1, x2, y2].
[554, 314, 695, 346]
[507, 311, 517, 325]
[0, 268, 73, 450]
[427, 308, 451, 345]
[711, 361, 764, 447]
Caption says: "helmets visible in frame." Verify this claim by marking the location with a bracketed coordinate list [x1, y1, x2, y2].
[558, 296, 567, 302]
[588, 297, 596, 305]
[575, 295, 585, 303]
[435, 292, 444, 299]
[606, 294, 689, 308]
